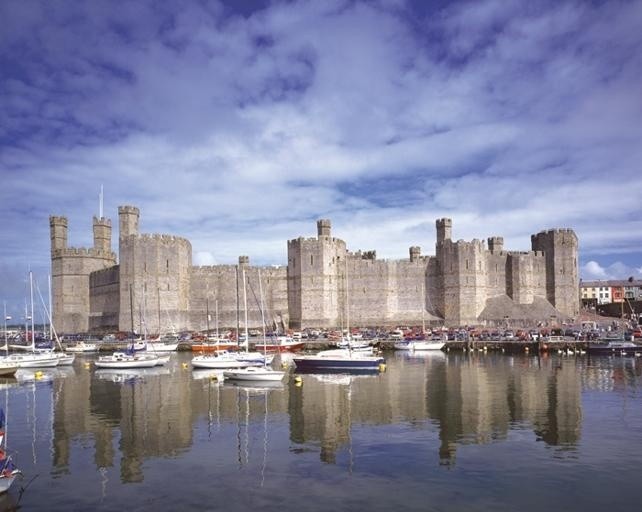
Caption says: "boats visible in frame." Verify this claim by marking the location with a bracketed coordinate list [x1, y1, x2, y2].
[0, 449, 24, 494]
[66, 341, 98, 352]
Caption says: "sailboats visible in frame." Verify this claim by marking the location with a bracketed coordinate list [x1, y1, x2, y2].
[190, 256, 307, 381]
[0, 271, 76, 376]
[292, 250, 387, 371]
[94, 284, 180, 368]
[393, 280, 448, 350]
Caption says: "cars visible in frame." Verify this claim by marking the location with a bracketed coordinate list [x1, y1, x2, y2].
[387, 327, 642, 340]
